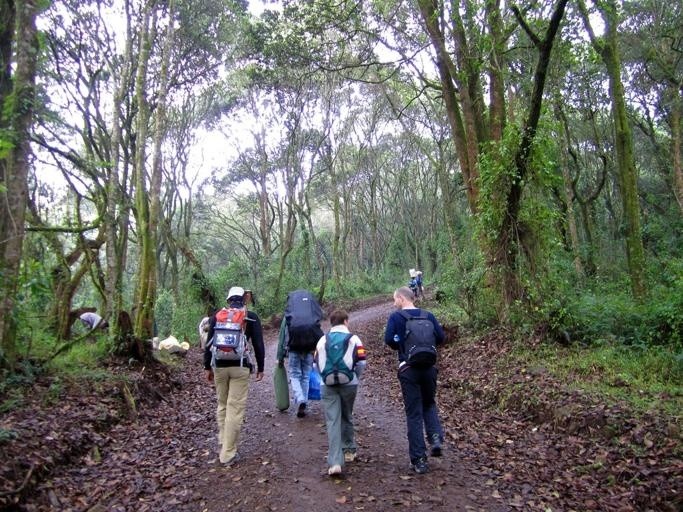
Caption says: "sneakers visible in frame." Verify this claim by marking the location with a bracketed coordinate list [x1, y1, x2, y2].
[220, 452, 240, 467]
[297, 400, 308, 418]
[430, 433, 443, 458]
[344, 451, 357, 463]
[328, 464, 342, 476]
[409, 457, 427, 475]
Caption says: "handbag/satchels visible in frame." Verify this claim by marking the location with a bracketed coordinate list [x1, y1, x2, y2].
[274, 366, 291, 412]
[308, 366, 322, 401]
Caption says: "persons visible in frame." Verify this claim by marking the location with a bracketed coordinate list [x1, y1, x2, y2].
[313, 310, 366, 476]
[276, 295, 325, 418]
[382, 286, 446, 474]
[409, 272, 425, 298]
[205, 287, 264, 465]
[80, 313, 107, 343]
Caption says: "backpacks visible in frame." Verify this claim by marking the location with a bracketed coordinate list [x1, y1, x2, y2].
[317, 330, 357, 388]
[206, 307, 259, 375]
[284, 290, 322, 352]
[395, 309, 437, 367]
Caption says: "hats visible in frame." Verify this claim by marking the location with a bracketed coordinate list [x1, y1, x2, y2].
[226, 286, 245, 301]
